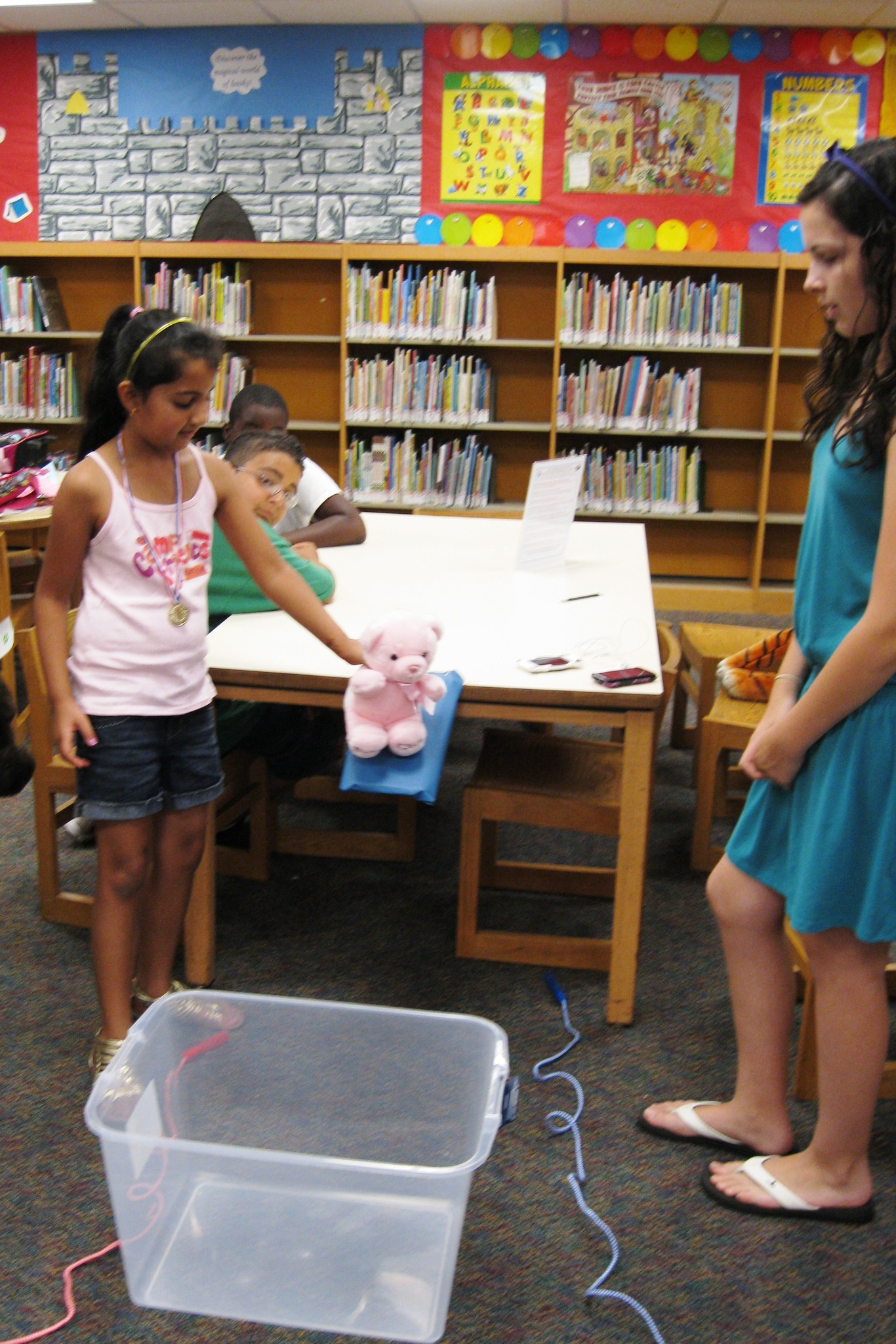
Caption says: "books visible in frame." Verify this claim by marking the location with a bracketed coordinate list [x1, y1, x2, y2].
[141, 260, 252, 334]
[557, 357, 699, 432]
[344, 262, 498, 340]
[0, 347, 79, 419]
[345, 430, 493, 508]
[555, 445, 703, 514]
[345, 348, 491, 422]
[560, 274, 743, 347]
[209, 353, 252, 423]
[0, 265, 69, 333]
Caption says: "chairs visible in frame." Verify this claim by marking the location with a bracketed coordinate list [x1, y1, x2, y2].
[0, 532, 415, 929]
[456, 620, 785, 974]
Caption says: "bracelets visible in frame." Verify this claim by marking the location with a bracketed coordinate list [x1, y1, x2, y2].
[774, 675, 804, 685]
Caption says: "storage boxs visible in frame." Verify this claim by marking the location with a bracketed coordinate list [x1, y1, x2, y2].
[83, 989, 522, 1344]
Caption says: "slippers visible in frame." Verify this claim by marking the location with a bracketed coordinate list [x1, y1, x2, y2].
[701, 1156, 876, 1224]
[638, 1098, 767, 1154]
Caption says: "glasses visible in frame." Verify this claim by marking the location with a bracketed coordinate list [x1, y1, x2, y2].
[234, 466, 297, 511]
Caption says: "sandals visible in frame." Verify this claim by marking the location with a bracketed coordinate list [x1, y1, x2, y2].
[86, 1027, 140, 1124]
[131, 978, 246, 1031]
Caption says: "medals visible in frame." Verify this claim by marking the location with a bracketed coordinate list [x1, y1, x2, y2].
[169, 604, 189, 626]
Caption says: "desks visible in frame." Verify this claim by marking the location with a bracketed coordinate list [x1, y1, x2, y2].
[204, 512, 666, 1023]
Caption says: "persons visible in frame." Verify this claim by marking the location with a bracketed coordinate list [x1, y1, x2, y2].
[644, 138, 896, 1224]
[33, 302, 368, 1120]
[64, 429, 337, 850]
[211, 385, 366, 548]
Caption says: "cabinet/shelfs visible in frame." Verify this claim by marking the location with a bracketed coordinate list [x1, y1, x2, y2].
[0, 241, 823, 588]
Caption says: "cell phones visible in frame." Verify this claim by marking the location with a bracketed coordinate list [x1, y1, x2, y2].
[528, 657, 579, 673]
[592, 668, 656, 687]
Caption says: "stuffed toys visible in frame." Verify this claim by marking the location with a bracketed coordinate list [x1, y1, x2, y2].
[715, 627, 795, 700]
[342, 618, 446, 758]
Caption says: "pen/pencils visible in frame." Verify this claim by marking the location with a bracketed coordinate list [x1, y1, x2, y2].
[565, 594, 600, 602]
